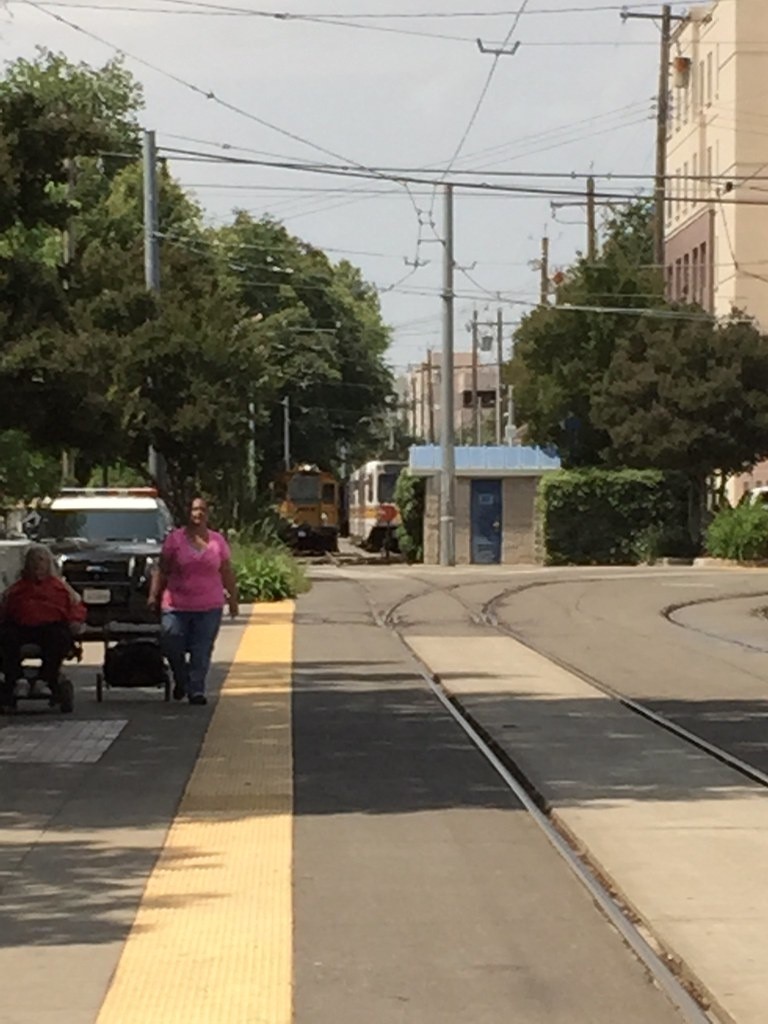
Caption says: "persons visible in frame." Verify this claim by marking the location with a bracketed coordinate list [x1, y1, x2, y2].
[0, 544, 87, 698]
[146, 497, 239, 705]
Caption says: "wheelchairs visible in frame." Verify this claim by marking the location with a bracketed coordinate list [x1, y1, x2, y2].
[0, 625, 83, 715]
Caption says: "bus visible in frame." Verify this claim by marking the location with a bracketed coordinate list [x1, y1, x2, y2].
[344, 460, 411, 552]
[269, 459, 346, 554]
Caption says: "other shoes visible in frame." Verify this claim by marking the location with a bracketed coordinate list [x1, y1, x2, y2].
[187, 694, 208, 705]
[174, 683, 185, 700]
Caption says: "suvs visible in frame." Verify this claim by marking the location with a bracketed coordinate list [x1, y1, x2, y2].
[27, 483, 176, 624]
